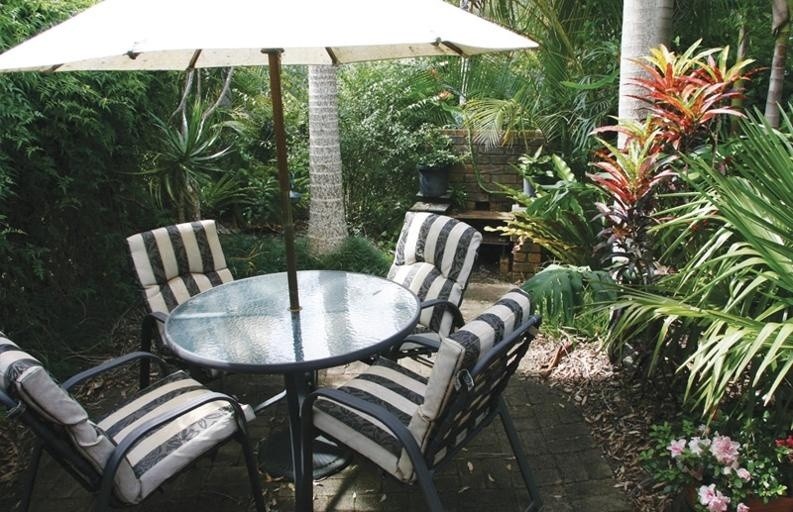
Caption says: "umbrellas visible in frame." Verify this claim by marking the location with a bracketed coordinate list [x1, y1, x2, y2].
[0, 0, 540, 311]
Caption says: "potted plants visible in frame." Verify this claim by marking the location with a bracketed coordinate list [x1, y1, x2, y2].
[518, 144, 554, 196]
[408, 122, 472, 197]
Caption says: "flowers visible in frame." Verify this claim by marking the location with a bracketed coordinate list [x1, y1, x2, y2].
[639, 420, 793, 512]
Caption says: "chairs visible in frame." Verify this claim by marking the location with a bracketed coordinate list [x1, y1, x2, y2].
[357, 211, 484, 366]
[1, 331, 266, 511]
[301, 286, 545, 512]
[124, 219, 315, 413]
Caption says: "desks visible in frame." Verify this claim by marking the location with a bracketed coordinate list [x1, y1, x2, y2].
[164, 270, 421, 482]
